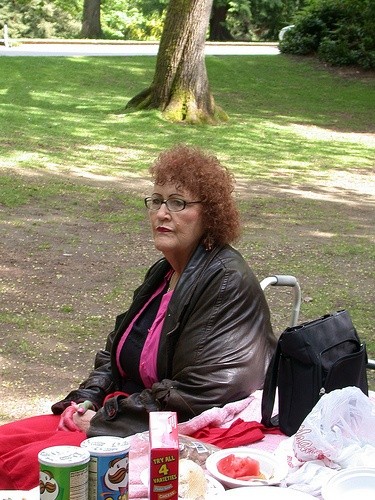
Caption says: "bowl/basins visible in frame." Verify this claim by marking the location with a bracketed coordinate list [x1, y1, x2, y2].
[323, 467, 375, 500]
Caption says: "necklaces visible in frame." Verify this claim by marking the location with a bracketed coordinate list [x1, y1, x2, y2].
[167, 272, 174, 292]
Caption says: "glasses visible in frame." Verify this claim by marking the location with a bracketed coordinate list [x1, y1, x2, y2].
[144, 195, 203, 213]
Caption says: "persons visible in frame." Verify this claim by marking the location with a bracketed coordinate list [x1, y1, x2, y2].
[0, 145, 278, 490]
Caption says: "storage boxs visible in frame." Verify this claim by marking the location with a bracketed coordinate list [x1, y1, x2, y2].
[148, 411, 178, 500]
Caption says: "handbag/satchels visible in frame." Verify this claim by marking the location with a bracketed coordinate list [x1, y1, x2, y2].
[260, 310, 370, 436]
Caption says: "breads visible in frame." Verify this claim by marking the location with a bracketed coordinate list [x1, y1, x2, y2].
[178, 434, 213, 465]
[178, 458, 207, 500]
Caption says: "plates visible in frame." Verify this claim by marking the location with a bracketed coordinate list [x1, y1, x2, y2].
[178, 473, 226, 500]
[205, 446, 289, 488]
[227, 485, 318, 500]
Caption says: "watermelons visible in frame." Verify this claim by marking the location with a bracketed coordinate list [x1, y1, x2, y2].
[216, 454, 260, 479]
